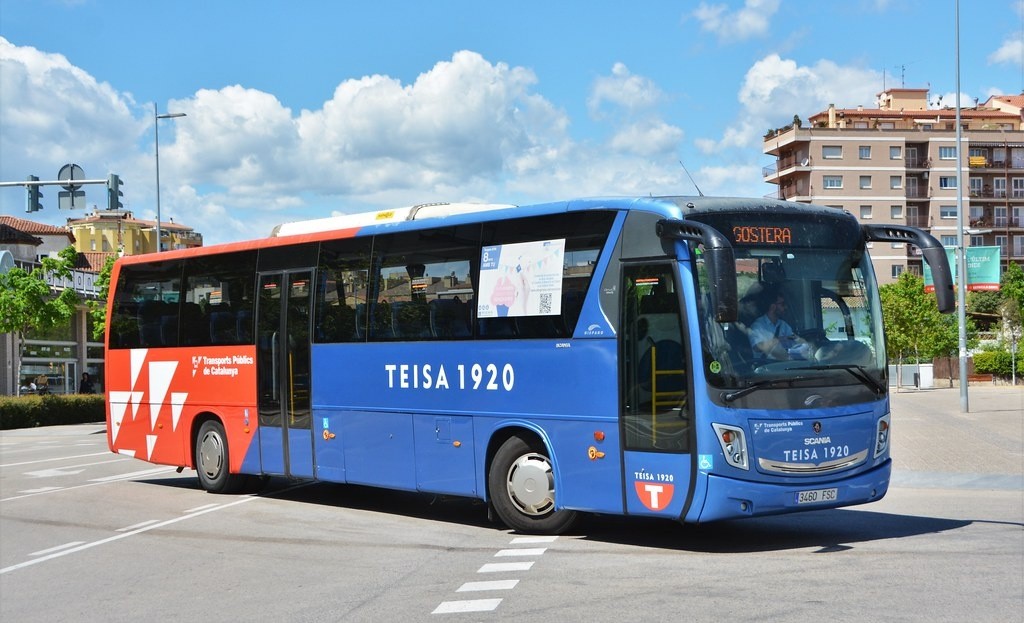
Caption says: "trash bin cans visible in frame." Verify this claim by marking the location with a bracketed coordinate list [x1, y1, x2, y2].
[918, 364, 934, 389]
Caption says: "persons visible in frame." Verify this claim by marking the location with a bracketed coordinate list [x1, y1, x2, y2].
[80, 372, 95, 394]
[748, 289, 816, 370]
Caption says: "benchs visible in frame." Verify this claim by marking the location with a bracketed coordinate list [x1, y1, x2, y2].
[134, 290, 585, 348]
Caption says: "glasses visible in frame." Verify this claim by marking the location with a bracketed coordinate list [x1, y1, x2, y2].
[776, 301, 785, 307]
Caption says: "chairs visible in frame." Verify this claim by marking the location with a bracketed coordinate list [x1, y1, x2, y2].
[737, 292, 768, 368]
[638, 293, 677, 315]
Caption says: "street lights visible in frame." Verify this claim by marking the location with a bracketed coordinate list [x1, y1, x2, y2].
[155, 102, 188, 300]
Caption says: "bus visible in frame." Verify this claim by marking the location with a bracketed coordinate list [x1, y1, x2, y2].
[104, 195, 957, 537]
